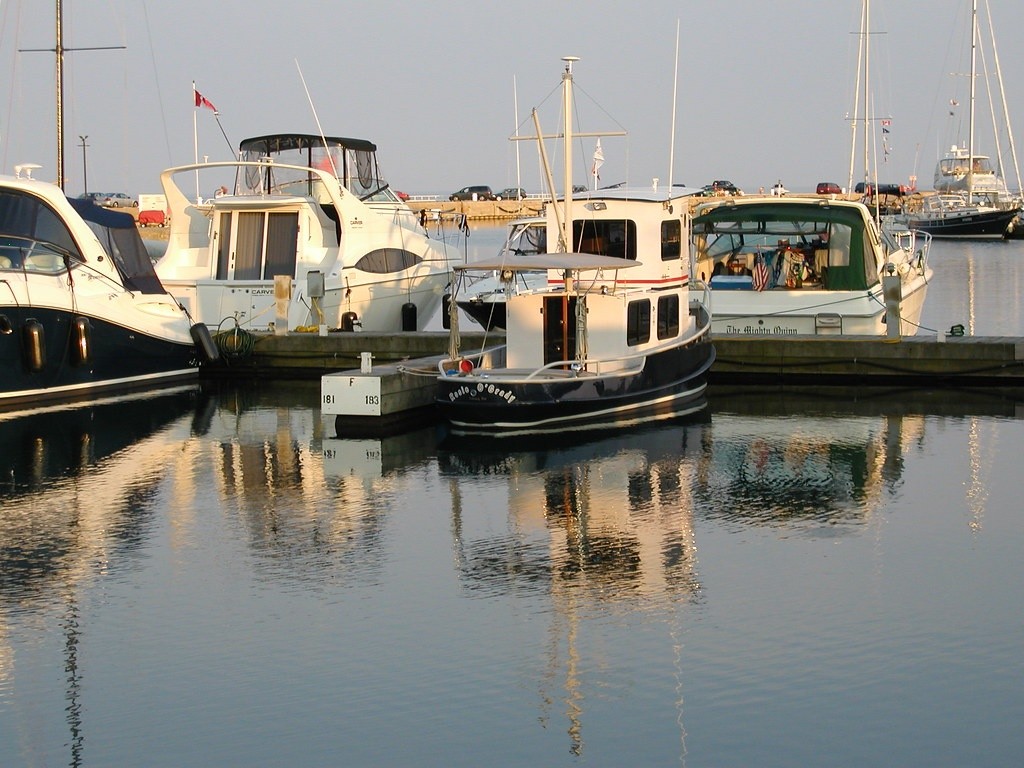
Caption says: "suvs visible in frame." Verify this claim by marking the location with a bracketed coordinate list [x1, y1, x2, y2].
[486, 187, 527, 202]
[448, 185, 492, 201]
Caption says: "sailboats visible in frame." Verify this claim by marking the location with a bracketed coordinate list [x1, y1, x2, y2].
[885, 0, 1024, 243]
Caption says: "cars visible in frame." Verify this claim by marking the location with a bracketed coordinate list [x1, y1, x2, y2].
[78, 192, 103, 201]
[93, 193, 139, 209]
[393, 191, 410, 202]
[854, 180, 905, 198]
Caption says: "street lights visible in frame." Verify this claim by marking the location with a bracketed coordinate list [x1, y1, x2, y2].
[76, 135, 92, 196]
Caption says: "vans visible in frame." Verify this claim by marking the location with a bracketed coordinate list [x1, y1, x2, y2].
[817, 182, 842, 194]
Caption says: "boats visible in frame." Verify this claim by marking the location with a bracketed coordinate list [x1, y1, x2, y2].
[930, 134, 1005, 190]
[688, 90, 934, 342]
[0, 162, 204, 425]
[136, 60, 487, 334]
[415, 15, 726, 439]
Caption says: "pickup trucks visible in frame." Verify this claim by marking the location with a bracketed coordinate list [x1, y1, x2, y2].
[701, 179, 742, 199]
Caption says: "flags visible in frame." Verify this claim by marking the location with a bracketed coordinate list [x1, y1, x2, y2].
[593, 139, 605, 179]
[900, 185, 915, 192]
[883, 128, 890, 133]
[883, 121, 890, 125]
[951, 100, 960, 106]
[195, 91, 217, 112]
[884, 137, 890, 162]
[752, 251, 769, 292]
